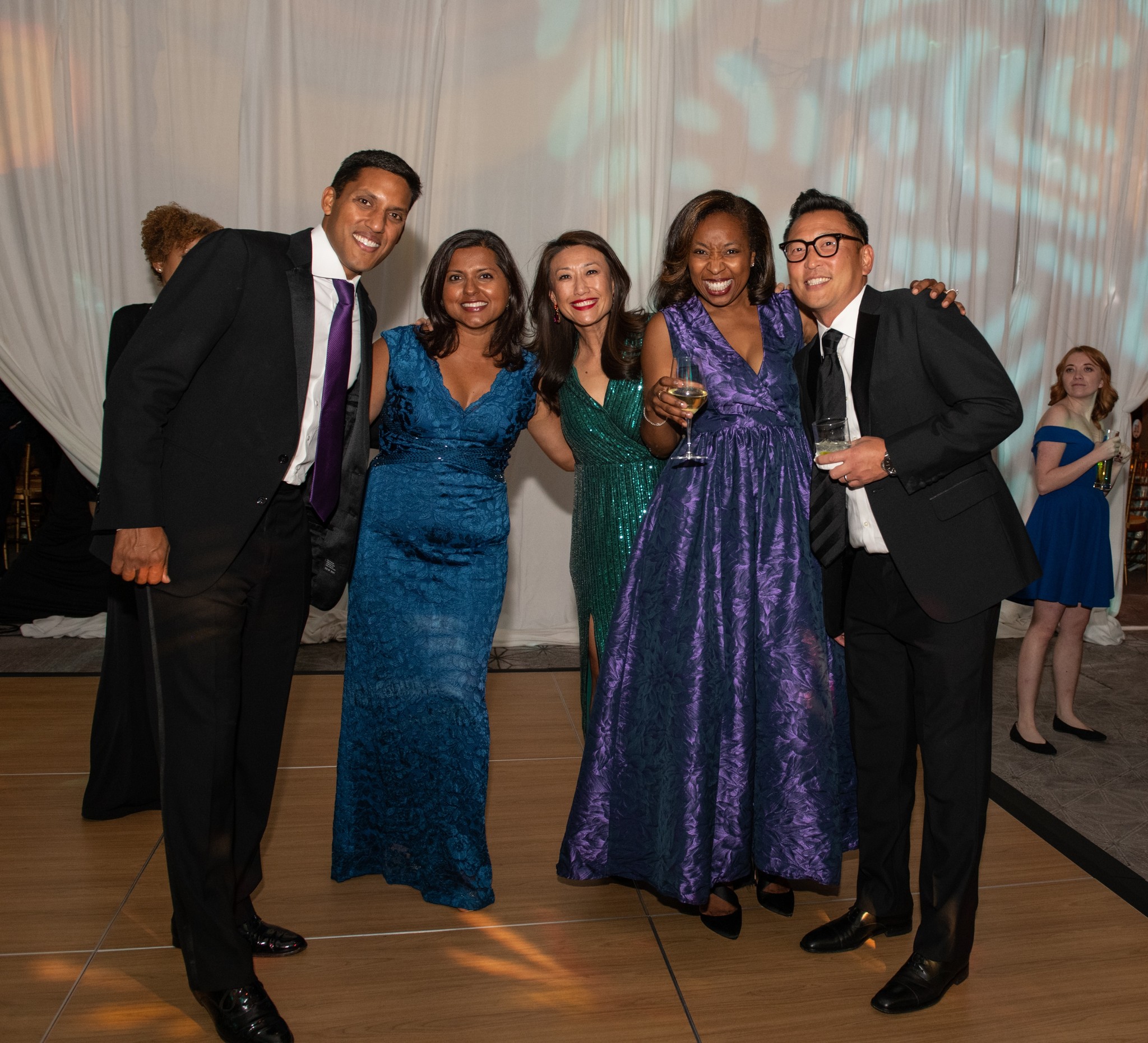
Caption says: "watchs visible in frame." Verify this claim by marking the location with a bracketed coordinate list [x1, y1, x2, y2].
[882, 450, 898, 476]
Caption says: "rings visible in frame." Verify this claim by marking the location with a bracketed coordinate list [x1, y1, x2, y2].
[844, 474, 853, 483]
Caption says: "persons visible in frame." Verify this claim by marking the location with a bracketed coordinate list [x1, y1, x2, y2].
[97, 149, 422, 1043]
[330, 229, 579, 909]
[526, 230, 665, 888]
[1006, 344, 1132, 755]
[777, 187, 1047, 1014]
[554, 187, 968, 941]
[81, 203, 225, 822]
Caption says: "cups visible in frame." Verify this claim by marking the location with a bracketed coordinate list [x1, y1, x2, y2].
[811, 416, 852, 470]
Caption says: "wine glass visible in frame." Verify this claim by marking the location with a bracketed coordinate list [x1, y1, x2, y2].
[666, 354, 711, 462]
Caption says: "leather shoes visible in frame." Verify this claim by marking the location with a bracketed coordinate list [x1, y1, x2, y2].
[195, 981, 295, 1043]
[1010, 721, 1057, 756]
[800, 904, 914, 951]
[1053, 713, 1108, 741]
[172, 913, 308, 956]
[871, 951, 969, 1015]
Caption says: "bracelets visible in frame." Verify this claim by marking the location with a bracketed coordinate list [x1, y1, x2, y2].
[643, 403, 668, 426]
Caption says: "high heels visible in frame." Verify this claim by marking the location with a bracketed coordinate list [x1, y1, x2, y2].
[756, 877, 795, 917]
[700, 885, 743, 939]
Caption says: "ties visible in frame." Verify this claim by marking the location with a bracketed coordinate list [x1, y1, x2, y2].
[809, 328, 850, 568]
[310, 279, 355, 523]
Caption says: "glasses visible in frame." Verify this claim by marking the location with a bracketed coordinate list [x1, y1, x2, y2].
[778, 233, 864, 263]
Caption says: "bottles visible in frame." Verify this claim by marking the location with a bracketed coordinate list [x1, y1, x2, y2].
[1093, 427, 1120, 491]
